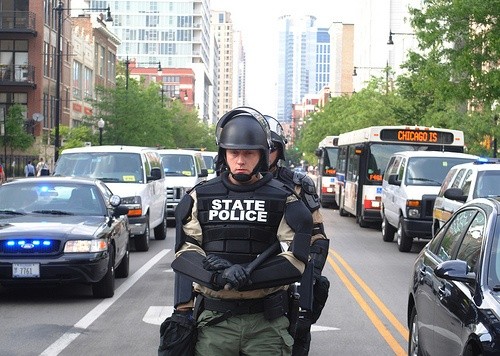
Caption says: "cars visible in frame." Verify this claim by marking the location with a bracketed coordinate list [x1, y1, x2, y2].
[294, 165, 317, 184]
[407, 194, 500, 356]
[0, 176, 132, 299]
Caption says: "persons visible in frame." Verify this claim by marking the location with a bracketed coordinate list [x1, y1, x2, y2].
[24, 160, 36, 177]
[174, 115, 331, 356]
[42, 160, 50, 174]
[158, 107, 312, 356]
[48, 158, 56, 174]
[0, 162, 6, 185]
[36, 157, 44, 171]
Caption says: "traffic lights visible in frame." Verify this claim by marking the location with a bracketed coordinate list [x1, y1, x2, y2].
[295, 145, 299, 152]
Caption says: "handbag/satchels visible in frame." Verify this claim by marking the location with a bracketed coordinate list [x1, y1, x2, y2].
[28, 173, 34, 176]
[157, 310, 198, 356]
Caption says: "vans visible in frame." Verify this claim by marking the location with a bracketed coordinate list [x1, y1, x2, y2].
[432, 156, 500, 238]
[380, 149, 485, 252]
[154, 149, 218, 226]
[40, 145, 168, 253]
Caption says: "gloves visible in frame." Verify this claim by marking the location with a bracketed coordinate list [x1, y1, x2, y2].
[202, 254, 230, 271]
[212, 264, 253, 292]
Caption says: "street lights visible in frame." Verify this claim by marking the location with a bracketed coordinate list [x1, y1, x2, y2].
[160, 87, 189, 110]
[352, 59, 389, 96]
[98, 117, 106, 146]
[52, 0, 114, 166]
[125, 56, 162, 91]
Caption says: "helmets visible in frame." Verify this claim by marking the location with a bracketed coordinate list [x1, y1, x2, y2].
[262, 115, 286, 162]
[215, 107, 270, 169]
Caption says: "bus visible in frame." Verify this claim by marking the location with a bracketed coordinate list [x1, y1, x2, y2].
[315, 136, 339, 209]
[334, 124, 468, 228]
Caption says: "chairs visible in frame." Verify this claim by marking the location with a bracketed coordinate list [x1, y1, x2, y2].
[5, 190, 37, 211]
[66, 186, 95, 213]
